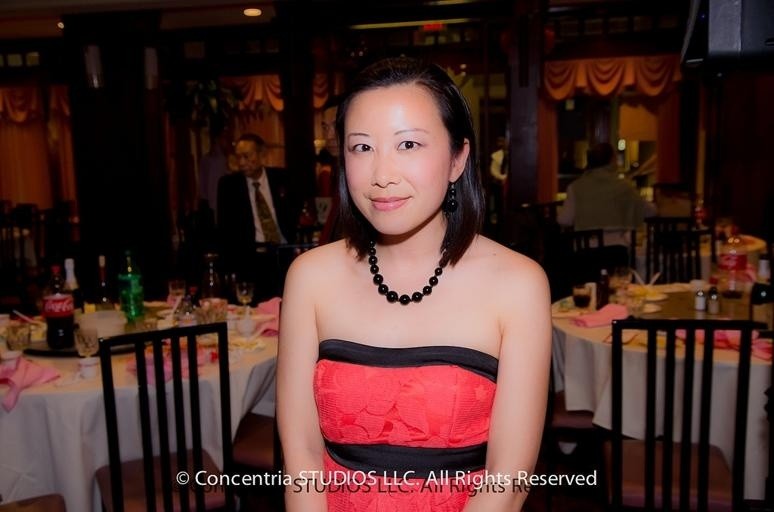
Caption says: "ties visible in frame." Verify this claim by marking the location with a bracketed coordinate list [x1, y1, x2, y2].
[253, 182, 276, 242]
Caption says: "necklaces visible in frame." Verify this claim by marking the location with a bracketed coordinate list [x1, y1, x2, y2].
[368, 238, 452, 305]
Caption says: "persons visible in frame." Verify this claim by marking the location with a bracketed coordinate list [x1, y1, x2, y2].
[214, 133, 304, 304]
[277, 55, 553, 512]
[555, 140, 662, 284]
[321, 95, 366, 244]
[482, 135, 508, 236]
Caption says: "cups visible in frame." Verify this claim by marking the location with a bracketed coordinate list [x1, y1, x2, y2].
[73, 327, 100, 358]
[169, 280, 186, 308]
[235, 318, 256, 337]
[572, 284, 593, 308]
[79, 359, 102, 381]
[199, 297, 229, 324]
[6, 321, 32, 350]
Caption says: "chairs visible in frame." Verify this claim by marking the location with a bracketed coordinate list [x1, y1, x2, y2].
[0, 197, 81, 316]
[549, 356, 610, 503]
[484, 181, 701, 285]
[0, 493, 67, 511]
[95, 320, 236, 512]
[598, 317, 753, 511]
[231, 300, 286, 512]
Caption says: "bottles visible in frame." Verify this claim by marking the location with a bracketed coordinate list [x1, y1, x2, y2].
[595, 261, 609, 310]
[114, 249, 148, 323]
[64, 255, 113, 305]
[43, 265, 77, 353]
[749, 252, 774, 339]
[694, 288, 720, 314]
[716, 224, 747, 299]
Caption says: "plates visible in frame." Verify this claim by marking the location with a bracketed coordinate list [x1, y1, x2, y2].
[156, 308, 190, 321]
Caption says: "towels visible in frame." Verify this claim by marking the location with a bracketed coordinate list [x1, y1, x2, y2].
[572, 301, 629, 328]
[1, 356, 62, 413]
[672, 317, 773, 361]
[255, 296, 284, 338]
[126, 347, 206, 387]
[719, 254, 756, 281]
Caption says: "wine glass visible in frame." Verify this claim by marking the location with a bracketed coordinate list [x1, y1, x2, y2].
[236, 283, 258, 312]
